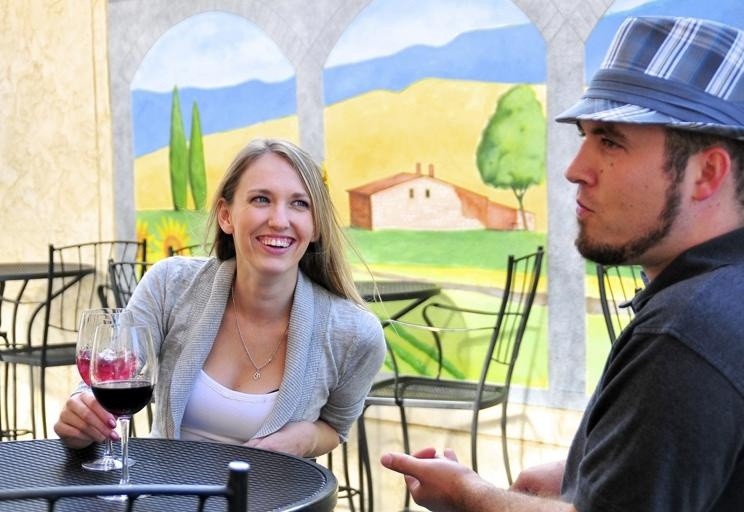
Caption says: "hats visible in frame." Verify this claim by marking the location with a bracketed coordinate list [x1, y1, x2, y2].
[556, 16, 744, 142]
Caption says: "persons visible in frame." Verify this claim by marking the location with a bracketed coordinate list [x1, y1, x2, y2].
[56, 136, 388, 460]
[380, 17, 744, 512]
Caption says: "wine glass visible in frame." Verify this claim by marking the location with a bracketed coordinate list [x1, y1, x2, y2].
[75, 306, 136, 472]
[89, 322, 159, 503]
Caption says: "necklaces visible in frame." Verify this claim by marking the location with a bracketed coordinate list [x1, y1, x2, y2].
[231, 282, 290, 382]
